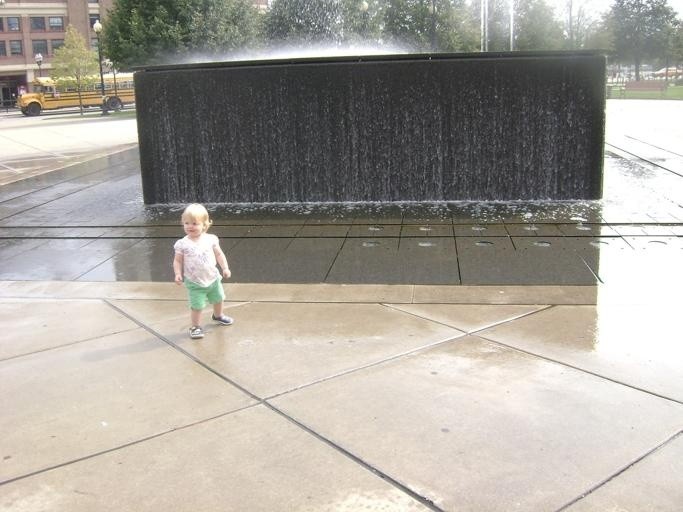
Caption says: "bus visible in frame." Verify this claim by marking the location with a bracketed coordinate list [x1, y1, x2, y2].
[15, 71, 137, 119]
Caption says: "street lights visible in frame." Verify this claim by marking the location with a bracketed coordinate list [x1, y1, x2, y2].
[108, 60, 117, 97]
[358, 0, 370, 37]
[35, 52, 46, 78]
[93, 19, 112, 118]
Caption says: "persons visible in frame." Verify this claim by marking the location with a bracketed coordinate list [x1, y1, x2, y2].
[171, 203, 234, 340]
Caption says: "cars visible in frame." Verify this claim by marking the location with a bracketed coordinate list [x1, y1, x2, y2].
[650, 67, 683, 80]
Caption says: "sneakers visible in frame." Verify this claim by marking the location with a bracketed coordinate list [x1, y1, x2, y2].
[211, 315, 233, 326]
[189, 326, 205, 338]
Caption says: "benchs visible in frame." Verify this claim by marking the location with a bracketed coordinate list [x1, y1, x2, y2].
[618, 79, 668, 99]
